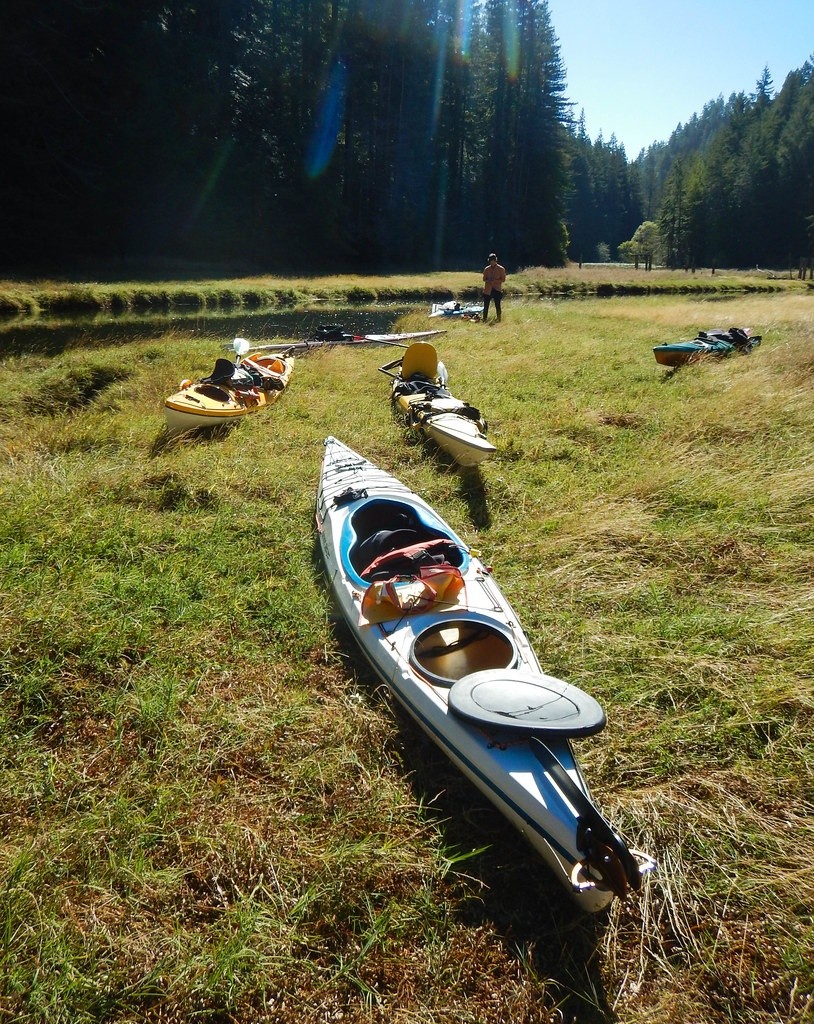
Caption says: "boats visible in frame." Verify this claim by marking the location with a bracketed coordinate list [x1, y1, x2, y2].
[651, 327, 763, 367]
[389, 341, 497, 468]
[222, 329, 447, 356]
[160, 344, 295, 431]
[427, 305, 484, 319]
[313, 432, 655, 915]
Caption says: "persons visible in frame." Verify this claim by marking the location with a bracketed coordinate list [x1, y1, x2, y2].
[482, 253, 506, 323]
